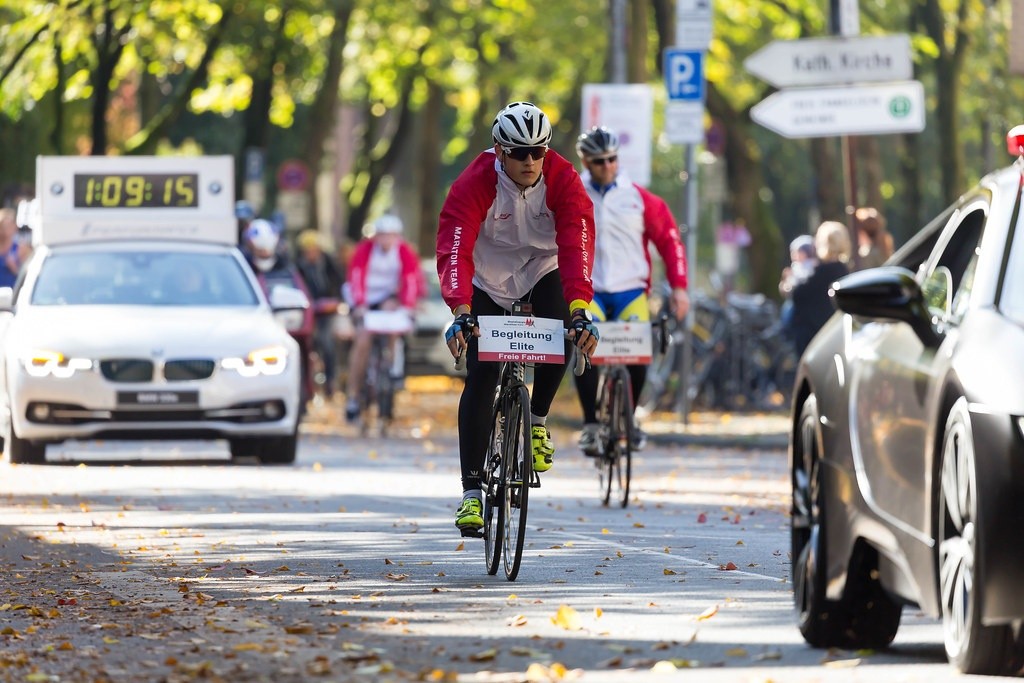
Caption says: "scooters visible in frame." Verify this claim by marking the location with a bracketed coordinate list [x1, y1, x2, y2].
[635, 274, 797, 423]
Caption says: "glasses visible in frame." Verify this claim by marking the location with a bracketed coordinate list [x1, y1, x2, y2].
[498, 142, 547, 161]
[585, 158, 619, 165]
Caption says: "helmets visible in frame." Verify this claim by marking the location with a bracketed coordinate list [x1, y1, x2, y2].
[302, 230, 327, 249]
[492, 101, 553, 148]
[575, 126, 618, 156]
[236, 200, 255, 221]
[248, 218, 279, 273]
[375, 215, 403, 234]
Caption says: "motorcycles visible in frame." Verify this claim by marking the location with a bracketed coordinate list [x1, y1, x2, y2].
[264, 284, 337, 426]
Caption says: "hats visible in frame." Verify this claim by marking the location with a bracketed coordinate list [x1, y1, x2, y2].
[817, 221, 851, 260]
[790, 236, 817, 258]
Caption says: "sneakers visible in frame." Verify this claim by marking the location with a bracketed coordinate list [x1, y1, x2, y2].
[454, 496, 486, 529]
[578, 425, 600, 450]
[531, 425, 553, 472]
[632, 422, 646, 452]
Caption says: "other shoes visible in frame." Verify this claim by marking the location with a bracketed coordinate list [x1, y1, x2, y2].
[345, 397, 360, 426]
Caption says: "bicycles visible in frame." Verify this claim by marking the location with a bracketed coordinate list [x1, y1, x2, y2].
[584, 312, 670, 509]
[350, 314, 417, 439]
[453, 319, 592, 581]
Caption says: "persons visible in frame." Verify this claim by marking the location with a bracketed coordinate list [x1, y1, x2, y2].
[717, 207, 895, 362]
[0, 208, 32, 288]
[435, 102, 600, 529]
[575, 126, 687, 453]
[235, 200, 427, 423]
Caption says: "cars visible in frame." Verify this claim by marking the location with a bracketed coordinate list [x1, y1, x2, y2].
[0, 241, 311, 465]
[786, 126, 1024, 677]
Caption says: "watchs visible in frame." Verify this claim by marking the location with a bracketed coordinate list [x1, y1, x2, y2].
[571, 309, 592, 322]
